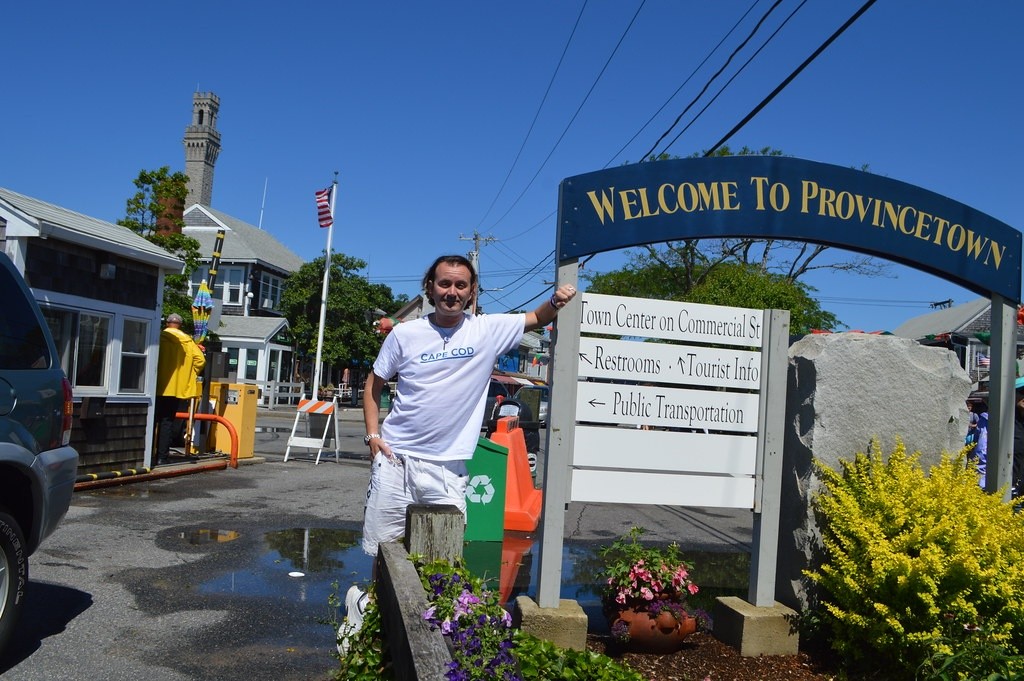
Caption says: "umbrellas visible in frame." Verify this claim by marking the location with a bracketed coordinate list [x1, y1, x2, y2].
[185, 280, 214, 455]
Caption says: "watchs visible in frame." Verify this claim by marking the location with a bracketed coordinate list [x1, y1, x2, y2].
[364, 432, 380, 445]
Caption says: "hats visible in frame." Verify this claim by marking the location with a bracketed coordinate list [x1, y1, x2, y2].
[166, 313, 183, 324]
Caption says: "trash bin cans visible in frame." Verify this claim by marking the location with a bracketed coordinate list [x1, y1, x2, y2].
[464, 436, 509, 589]
[380, 385, 391, 409]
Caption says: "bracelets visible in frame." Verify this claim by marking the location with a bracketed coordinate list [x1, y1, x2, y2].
[550, 294, 561, 310]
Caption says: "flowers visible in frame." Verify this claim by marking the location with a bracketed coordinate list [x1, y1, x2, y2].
[597, 527, 699, 600]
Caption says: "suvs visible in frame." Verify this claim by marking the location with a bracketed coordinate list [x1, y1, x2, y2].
[0, 250, 79, 654]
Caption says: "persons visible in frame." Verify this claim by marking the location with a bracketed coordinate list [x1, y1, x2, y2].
[967, 386, 1024, 512]
[335, 254, 576, 664]
[155, 313, 206, 463]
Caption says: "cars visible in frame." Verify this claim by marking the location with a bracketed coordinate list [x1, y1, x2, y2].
[513, 386, 549, 428]
[481, 377, 512, 432]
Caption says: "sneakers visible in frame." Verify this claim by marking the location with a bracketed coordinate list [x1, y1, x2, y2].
[336, 585, 371, 658]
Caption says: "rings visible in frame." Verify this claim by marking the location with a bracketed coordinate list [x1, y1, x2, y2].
[568, 287, 573, 291]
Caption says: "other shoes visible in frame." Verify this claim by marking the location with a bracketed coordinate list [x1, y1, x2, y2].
[155, 452, 170, 465]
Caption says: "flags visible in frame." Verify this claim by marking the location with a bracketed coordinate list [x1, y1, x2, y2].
[315, 185, 334, 227]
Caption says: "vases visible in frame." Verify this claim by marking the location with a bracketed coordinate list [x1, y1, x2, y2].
[604, 590, 695, 649]
